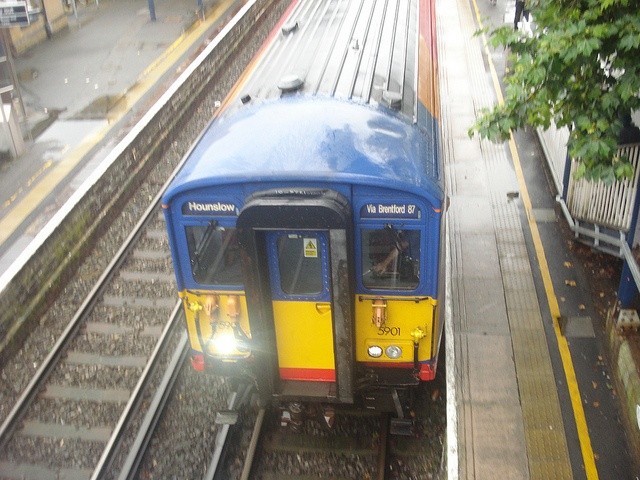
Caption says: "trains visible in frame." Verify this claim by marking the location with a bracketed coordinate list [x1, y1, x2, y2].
[162, 0, 450, 437]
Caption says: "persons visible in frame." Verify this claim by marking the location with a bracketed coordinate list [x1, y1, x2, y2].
[372, 231, 420, 276]
